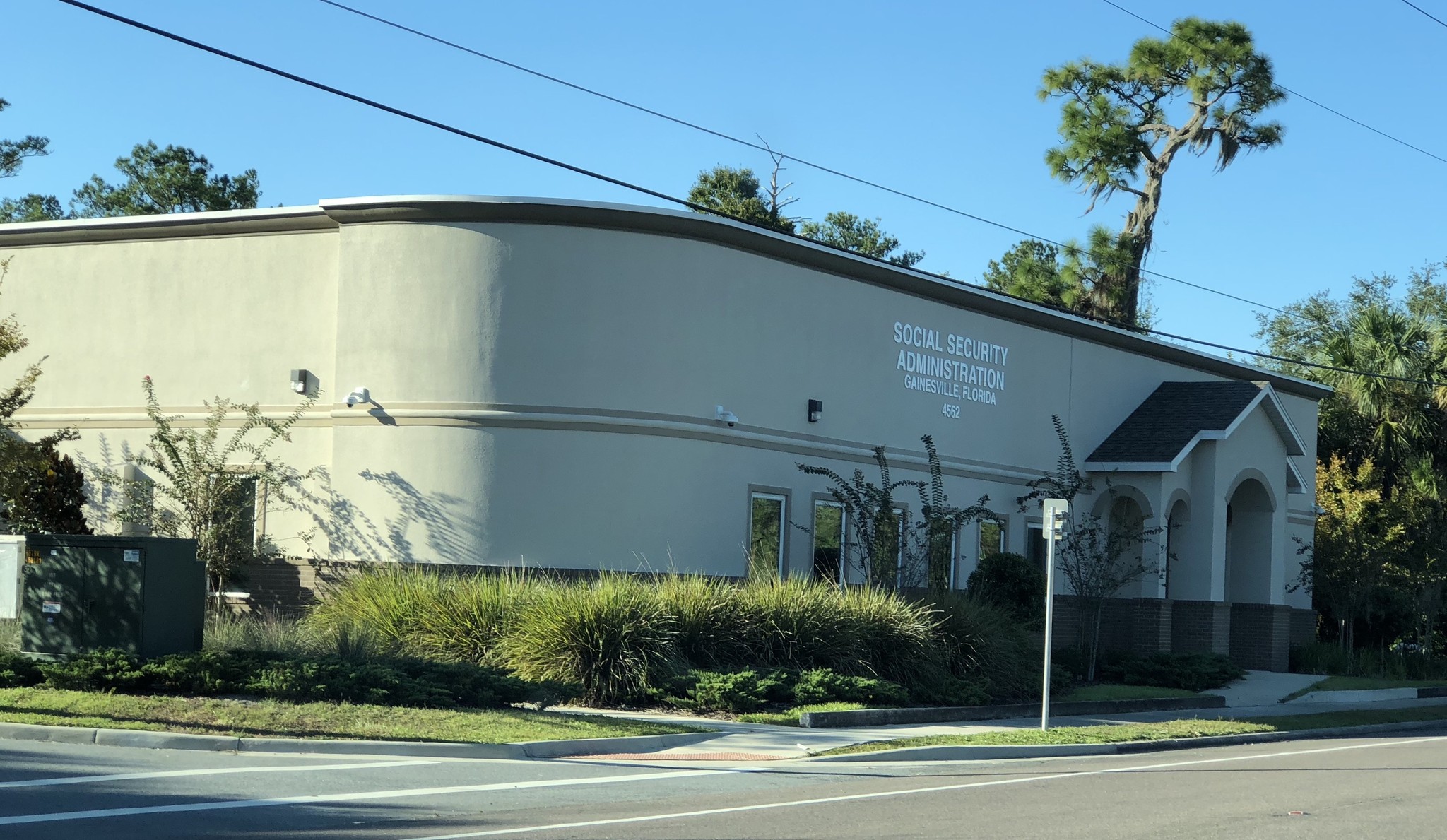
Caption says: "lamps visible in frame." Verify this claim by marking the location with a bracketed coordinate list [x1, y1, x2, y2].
[291, 369, 307, 393]
[808, 399, 822, 423]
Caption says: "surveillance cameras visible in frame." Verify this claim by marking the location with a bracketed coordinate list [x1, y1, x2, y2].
[1317, 509, 1326, 516]
[342, 396, 358, 408]
[723, 415, 739, 427]
